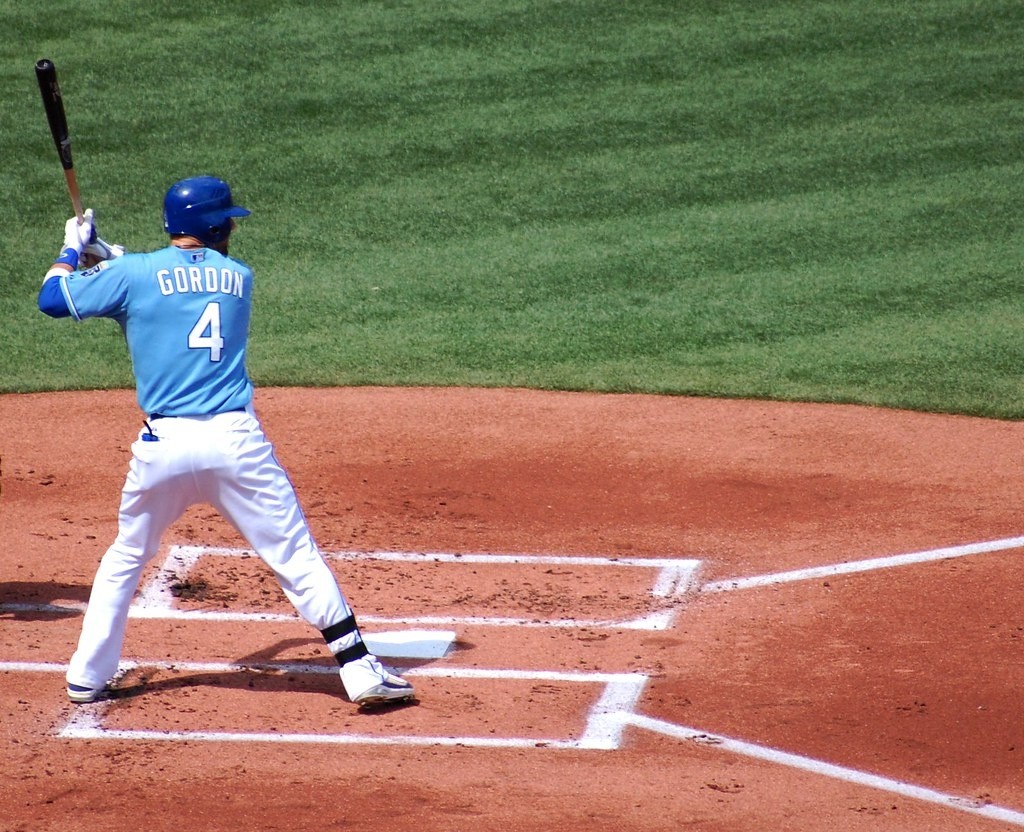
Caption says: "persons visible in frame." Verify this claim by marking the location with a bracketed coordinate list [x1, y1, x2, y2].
[38, 175, 415, 708]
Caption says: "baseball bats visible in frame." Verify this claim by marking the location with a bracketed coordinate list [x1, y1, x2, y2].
[34, 58, 85, 224]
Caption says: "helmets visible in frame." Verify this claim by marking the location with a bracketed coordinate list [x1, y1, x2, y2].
[162, 176, 251, 247]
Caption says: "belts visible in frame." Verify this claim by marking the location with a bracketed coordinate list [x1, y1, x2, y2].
[149, 405, 247, 421]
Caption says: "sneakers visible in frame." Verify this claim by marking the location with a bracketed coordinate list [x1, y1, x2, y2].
[355, 671, 415, 704]
[67, 683, 98, 702]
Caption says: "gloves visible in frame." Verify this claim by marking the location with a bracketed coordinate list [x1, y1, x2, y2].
[78, 236, 126, 269]
[60, 207, 93, 257]
